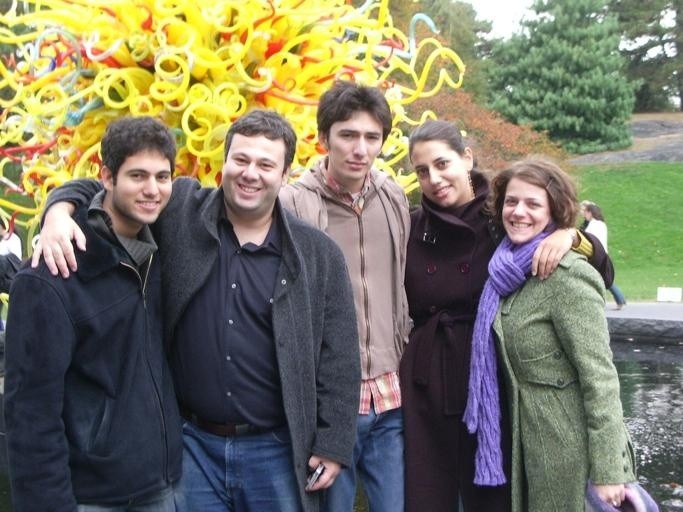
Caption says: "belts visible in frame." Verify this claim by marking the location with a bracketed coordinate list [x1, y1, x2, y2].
[181, 411, 268, 435]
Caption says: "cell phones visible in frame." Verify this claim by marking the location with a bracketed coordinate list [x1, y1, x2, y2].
[305, 462, 325, 491]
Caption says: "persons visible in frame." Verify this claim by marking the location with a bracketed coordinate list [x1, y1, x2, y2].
[489, 158, 636, 511]
[0, 212, 22, 331]
[0, 114, 187, 512]
[273, 80, 411, 512]
[579, 199, 597, 232]
[583, 204, 627, 311]
[399, 118, 615, 511]
[28, 110, 357, 512]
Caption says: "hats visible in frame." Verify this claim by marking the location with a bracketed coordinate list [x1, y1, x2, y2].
[585, 478, 659, 512]
